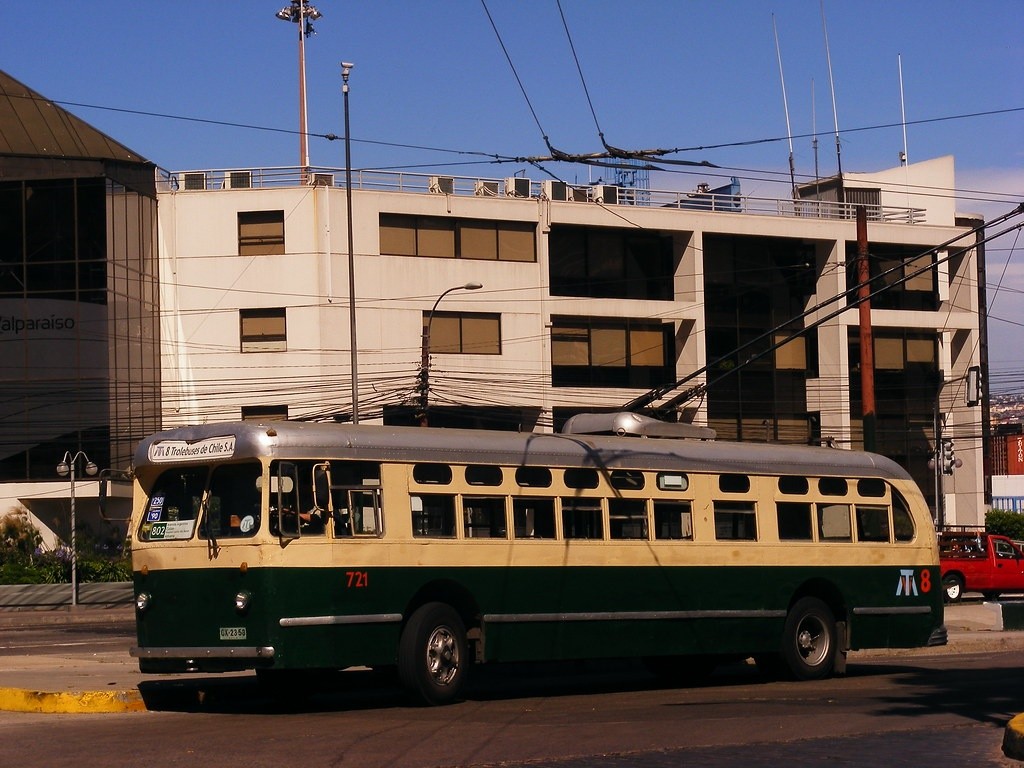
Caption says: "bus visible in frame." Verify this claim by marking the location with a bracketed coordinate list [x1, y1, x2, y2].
[98, 201, 1024, 716]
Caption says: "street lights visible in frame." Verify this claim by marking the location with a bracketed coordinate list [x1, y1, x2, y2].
[56, 450, 98, 606]
[275, 0, 323, 186]
[419, 281, 483, 427]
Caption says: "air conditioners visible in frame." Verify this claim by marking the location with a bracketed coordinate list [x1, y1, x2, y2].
[307, 170, 334, 187]
[593, 186, 620, 206]
[225, 171, 253, 189]
[475, 181, 499, 197]
[503, 177, 532, 198]
[429, 176, 455, 195]
[540, 179, 568, 201]
[568, 188, 589, 205]
[178, 173, 208, 190]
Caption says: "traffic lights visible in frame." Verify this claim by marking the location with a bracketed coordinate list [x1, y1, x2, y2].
[940, 438, 956, 476]
[964, 365, 983, 408]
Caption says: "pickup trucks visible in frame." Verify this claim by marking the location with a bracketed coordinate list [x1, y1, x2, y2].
[939, 534, 1024, 604]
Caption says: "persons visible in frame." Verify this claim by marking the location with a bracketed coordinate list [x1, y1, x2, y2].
[283, 482, 324, 533]
